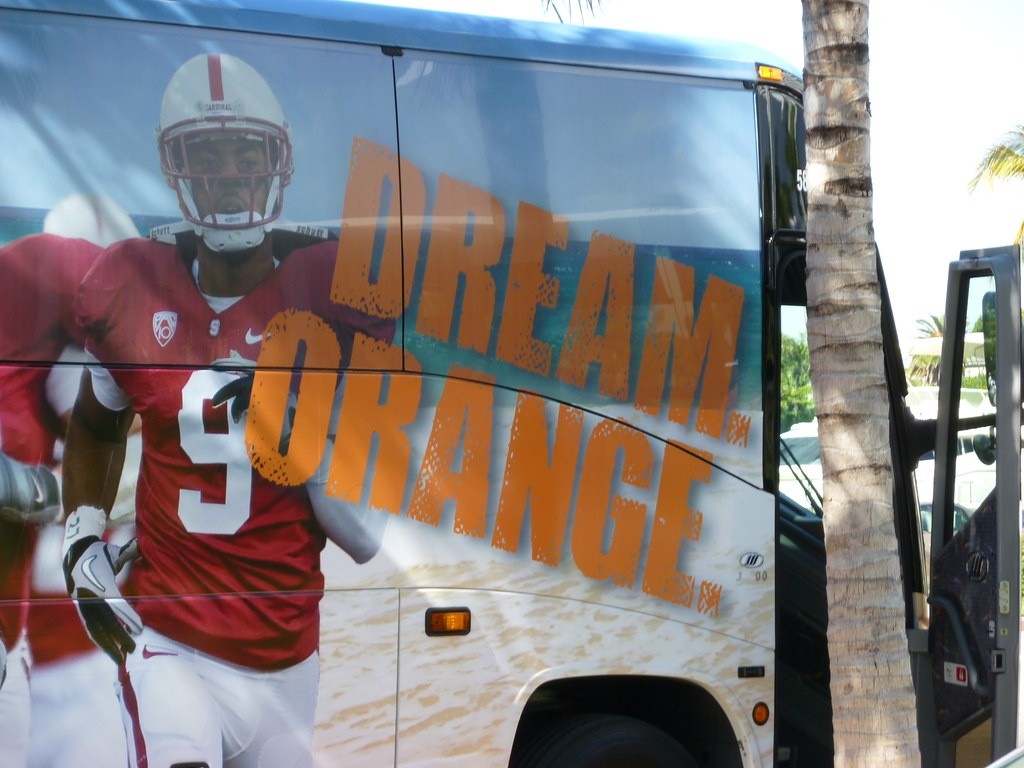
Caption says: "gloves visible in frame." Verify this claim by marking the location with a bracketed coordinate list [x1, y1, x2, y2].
[208, 349, 298, 450]
[60, 504, 142, 666]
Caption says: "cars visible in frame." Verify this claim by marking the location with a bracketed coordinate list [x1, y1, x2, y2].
[779, 401, 997, 517]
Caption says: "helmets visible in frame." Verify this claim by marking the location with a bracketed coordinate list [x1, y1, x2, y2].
[159, 52, 293, 254]
[42, 191, 142, 250]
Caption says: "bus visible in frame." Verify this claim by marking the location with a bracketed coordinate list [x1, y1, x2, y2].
[0, 1, 1024, 768]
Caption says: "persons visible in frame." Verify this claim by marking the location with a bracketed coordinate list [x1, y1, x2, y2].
[0, 54, 396, 768]
